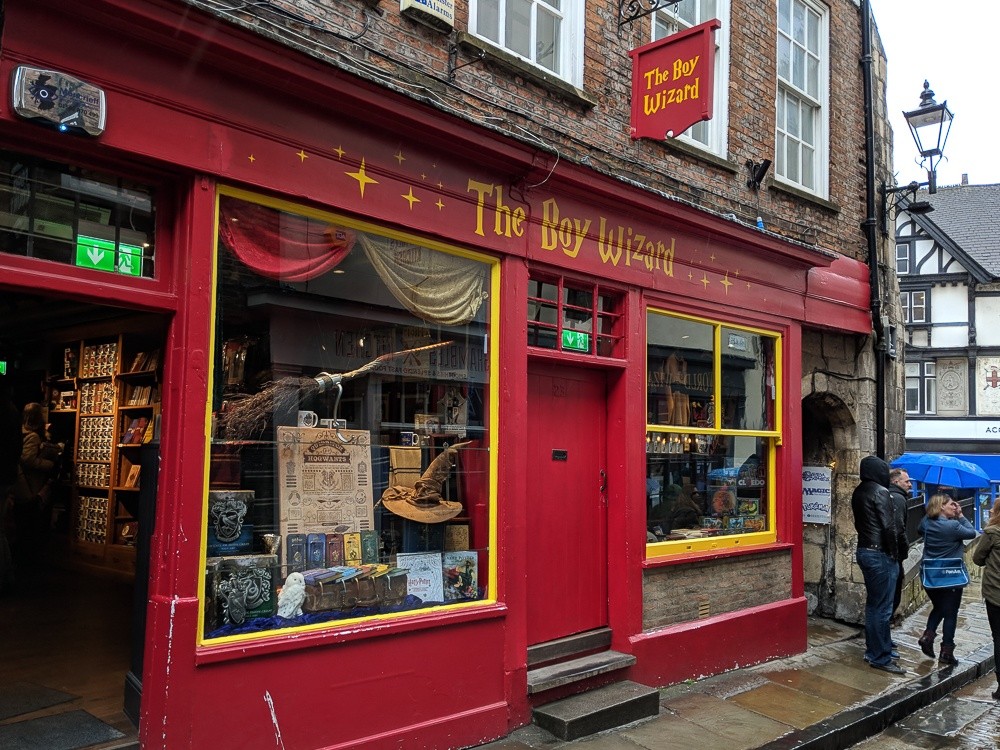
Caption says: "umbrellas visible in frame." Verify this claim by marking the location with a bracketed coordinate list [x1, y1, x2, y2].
[888, 452, 993, 494]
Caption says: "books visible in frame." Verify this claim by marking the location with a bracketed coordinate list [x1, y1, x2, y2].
[124, 465, 141, 487]
[120, 417, 153, 444]
[130, 349, 162, 372]
[127, 384, 161, 406]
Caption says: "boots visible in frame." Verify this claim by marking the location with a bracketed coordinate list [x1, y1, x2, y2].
[939, 643, 958, 665]
[918, 628, 937, 658]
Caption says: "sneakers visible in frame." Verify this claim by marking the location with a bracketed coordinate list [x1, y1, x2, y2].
[869, 658, 906, 675]
[864, 650, 900, 662]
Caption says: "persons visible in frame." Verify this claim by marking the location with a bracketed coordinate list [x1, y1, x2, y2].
[915, 493, 976, 665]
[972, 497, 1000, 700]
[852, 455, 912, 674]
[13, 402, 56, 570]
[649, 482, 703, 536]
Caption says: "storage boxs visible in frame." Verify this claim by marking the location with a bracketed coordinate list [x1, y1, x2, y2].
[204, 552, 279, 636]
[699, 484, 765, 537]
[396, 550, 479, 604]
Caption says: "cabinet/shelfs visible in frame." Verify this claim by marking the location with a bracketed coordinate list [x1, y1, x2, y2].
[43, 311, 166, 587]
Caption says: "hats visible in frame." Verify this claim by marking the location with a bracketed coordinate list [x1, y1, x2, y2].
[382, 441, 473, 523]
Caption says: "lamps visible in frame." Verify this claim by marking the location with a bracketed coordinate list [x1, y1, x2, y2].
[565, 288, 593, 321]
[877, 79, 954, 239]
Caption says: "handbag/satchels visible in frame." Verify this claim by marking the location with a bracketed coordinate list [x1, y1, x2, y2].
[922, 558, 969, 590]
